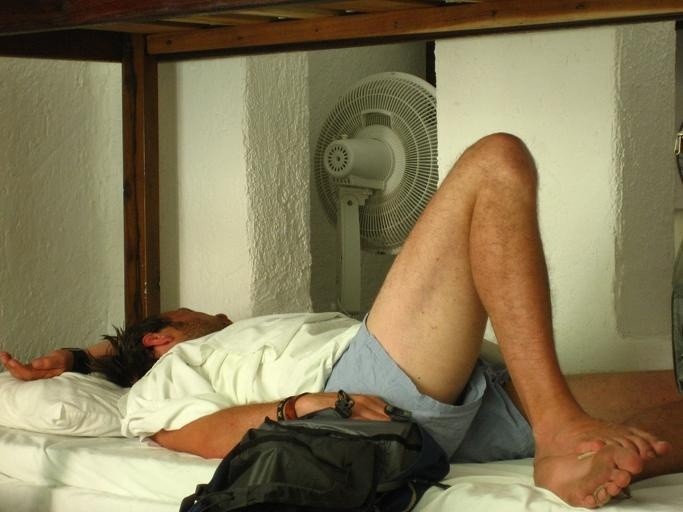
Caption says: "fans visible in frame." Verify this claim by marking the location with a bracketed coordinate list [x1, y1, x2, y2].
[314, 71, 439, 313]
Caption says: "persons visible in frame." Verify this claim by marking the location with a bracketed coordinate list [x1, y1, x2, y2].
[0, 133, 683, 509]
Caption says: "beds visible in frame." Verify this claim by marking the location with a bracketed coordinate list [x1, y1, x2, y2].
[0, 0, 682, 512]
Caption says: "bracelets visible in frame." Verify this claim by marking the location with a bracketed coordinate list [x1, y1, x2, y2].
[277, 396, 294, 423]
[284, 392, 310, 421]
[61, 347, 90, 372]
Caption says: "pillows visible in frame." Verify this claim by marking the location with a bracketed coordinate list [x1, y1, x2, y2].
[0, 368, 131, 436]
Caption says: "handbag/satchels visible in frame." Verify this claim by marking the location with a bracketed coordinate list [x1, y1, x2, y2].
[180, 391, 450, 511]
